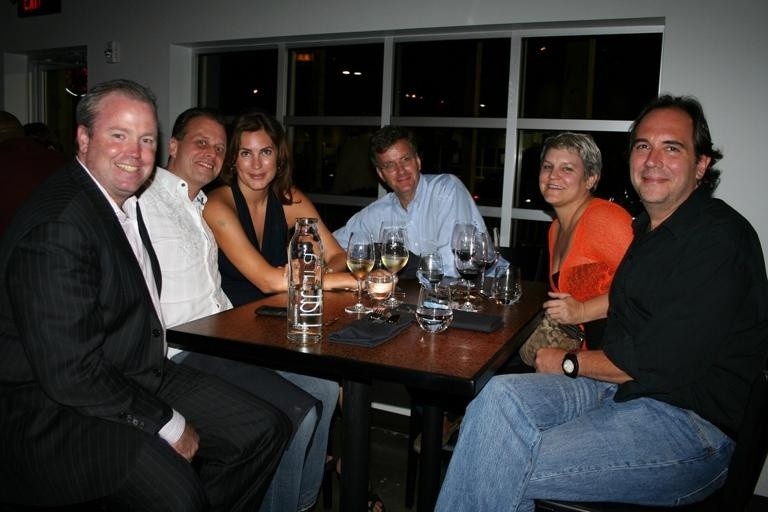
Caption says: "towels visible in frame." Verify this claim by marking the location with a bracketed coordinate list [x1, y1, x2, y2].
[328, 313, 409, 349]
[391, 301, 504, 333]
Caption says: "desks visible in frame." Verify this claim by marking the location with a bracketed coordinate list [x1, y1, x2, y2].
[166, 279, 555, 512]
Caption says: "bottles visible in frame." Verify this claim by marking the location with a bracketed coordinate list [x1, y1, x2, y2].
[285, 217, 326, 347]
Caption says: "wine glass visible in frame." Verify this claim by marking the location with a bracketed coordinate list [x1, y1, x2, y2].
[343, 232, 375, 316]
[449, 222, 497, 313]
[378, 220, 407, 308]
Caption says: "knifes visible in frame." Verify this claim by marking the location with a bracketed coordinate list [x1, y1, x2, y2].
[383, 313, 405, 327]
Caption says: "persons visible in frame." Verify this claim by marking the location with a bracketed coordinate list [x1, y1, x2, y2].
[135, 105, 340, 511]
[433, 93, 768, 511]
[204, 112, 386, 511]
[415, 130, 635, 454]
[0, 78, 293, 511]
[334, 125, 494, 279]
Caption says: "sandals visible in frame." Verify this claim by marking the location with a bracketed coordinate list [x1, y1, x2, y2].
[336, 459, 384, 511]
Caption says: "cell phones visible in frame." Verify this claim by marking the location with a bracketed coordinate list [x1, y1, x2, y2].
[254, 305, 287, 317]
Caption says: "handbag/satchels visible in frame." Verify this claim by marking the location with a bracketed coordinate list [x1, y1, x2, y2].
[519, 316, 585, 371]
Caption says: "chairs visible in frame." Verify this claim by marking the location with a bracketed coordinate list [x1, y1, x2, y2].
[532, 374, 768, 512]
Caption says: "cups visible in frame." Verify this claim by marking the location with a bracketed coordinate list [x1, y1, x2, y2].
[417, 252, 445, 290]
[414, 283, 454, 334]
[365, 258, 391, 301]
[491, 265, 523, 306]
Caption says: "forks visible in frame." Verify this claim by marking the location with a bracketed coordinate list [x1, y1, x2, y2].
[368, 307, 387, 322]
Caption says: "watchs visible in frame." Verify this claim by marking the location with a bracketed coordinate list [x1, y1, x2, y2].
[562, 347, 579, 378]
